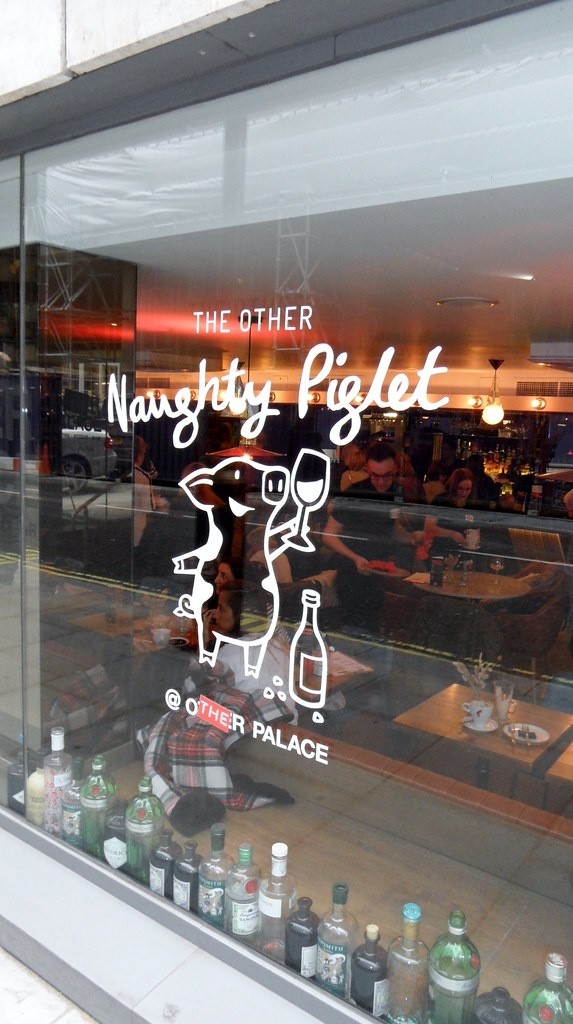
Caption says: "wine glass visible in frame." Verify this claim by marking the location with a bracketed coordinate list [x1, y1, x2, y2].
[144, 599, 195, 640]
[444, 552, 505, 585]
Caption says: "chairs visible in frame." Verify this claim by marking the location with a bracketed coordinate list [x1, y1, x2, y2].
[235, 528, 569, 705]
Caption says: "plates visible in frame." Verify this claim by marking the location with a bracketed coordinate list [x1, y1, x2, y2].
[461, 717, 550, 746]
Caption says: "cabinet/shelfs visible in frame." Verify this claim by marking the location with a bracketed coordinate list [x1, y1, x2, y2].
[424, 414, 546, 514]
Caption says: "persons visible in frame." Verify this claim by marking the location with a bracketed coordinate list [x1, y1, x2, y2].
[133, 437, 572, 727]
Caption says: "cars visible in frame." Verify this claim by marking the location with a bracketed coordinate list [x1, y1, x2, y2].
[56, 425, 118, 495]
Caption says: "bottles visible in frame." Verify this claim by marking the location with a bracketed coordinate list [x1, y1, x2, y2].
[317, 883, 358, 999]
[7, 727, 72, 839]
[313, 634, 331, 687]
[522, 952, 573, 1024]
[382, 902, 429, 1024]
[60, 756, 86, 848]
[104, 799, 129, 874]
[125, 776, 165, 887]
[368, 409, 543, 516]
[172, 840, 204, 914]
[285, 897, 320, 983]
[471, 987, 522, 1024]
[81, 754, 118, 863]
[430, 556, 444, 588]
[224, 843, 261, 950]
[148, 829, 184, 900]
[428, 911, 481, 1024]
[254, 843, 297, 966]
[350, 924, 388, 1019]
[196, 824, 236, 935]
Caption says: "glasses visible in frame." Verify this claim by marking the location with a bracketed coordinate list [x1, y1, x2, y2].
[367, 470, 393, 481]
[396, 471, 408, 477]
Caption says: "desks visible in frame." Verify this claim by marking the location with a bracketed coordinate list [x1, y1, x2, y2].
[391, 682, 573, 820]
[410, 571, 533, 606]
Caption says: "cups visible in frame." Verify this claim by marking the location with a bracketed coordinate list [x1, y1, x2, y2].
[462, 701, 494, 720]
[150, 628, 170, 650]
[495, 681, 515, 725]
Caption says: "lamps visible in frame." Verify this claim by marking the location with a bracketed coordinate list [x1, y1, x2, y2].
[531, 397, 547, 410]
[308, 393, 321, 403]
[206, 317, 287, 461]
[355, 394, 367, 404]
[468, 396, 483, 408]
[147, 390, 160, 399]
[482, 358, 505, 425]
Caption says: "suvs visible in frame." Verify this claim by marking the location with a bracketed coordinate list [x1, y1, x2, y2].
[95, 394, 136, 476]
